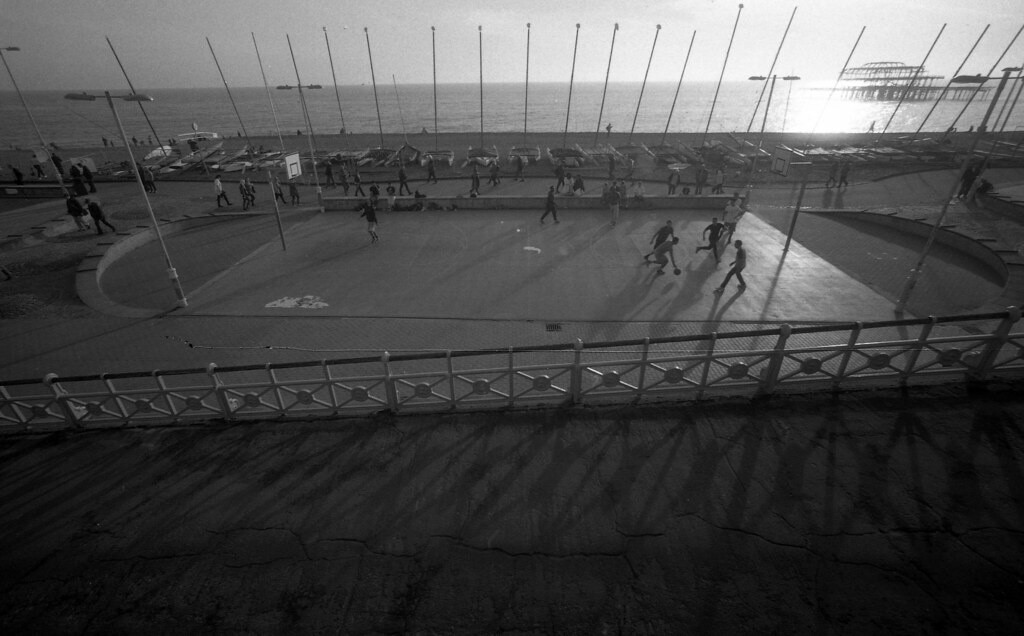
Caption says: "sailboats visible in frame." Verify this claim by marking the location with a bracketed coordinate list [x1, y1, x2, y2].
[105, 4, 1024, 178]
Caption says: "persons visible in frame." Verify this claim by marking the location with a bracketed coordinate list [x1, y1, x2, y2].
[76, 163, 96, 193]
[718, 198, 742, 243]
[69, 165, 88, 194]
[30, 153, 47, 179]
[8, 164, 23, 185]
[50, 153, 63, 176]
[360, 200, 379, 242]
[695, 217, 726, 263]
[646, 236, 678, 274]
[64, 193, 90, 231]
[540, 185, 558, 223]
[606, 186, 621, 227]
[644, 219, 674, 260]
[84, 198, 115, 235]
[7, 143, 21, 150]
[715, 240, 746, 290]
[96, 119, 992, 210]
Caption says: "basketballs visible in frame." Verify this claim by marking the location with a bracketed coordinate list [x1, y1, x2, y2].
[673, 268, 682, 275]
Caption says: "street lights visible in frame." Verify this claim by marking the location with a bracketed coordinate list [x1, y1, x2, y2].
[0, 45, 70, 198]
[895, 68, 1024, 313]
[63, 91, 188, 306]
[746, 75, 799, 201]
